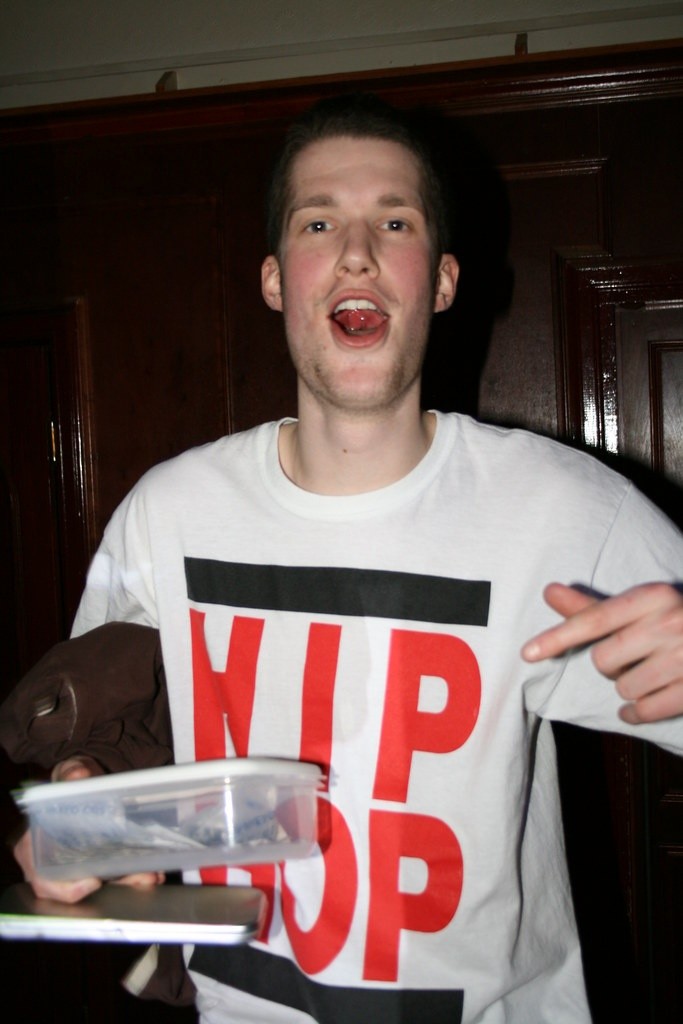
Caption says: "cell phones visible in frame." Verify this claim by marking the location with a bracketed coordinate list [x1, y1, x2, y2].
[0, 882, 267, 944]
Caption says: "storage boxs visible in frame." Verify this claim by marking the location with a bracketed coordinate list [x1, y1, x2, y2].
[16, 756, 327, 882]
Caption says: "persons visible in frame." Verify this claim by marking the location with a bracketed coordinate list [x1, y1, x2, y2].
[14, 96, 683, 1024]
